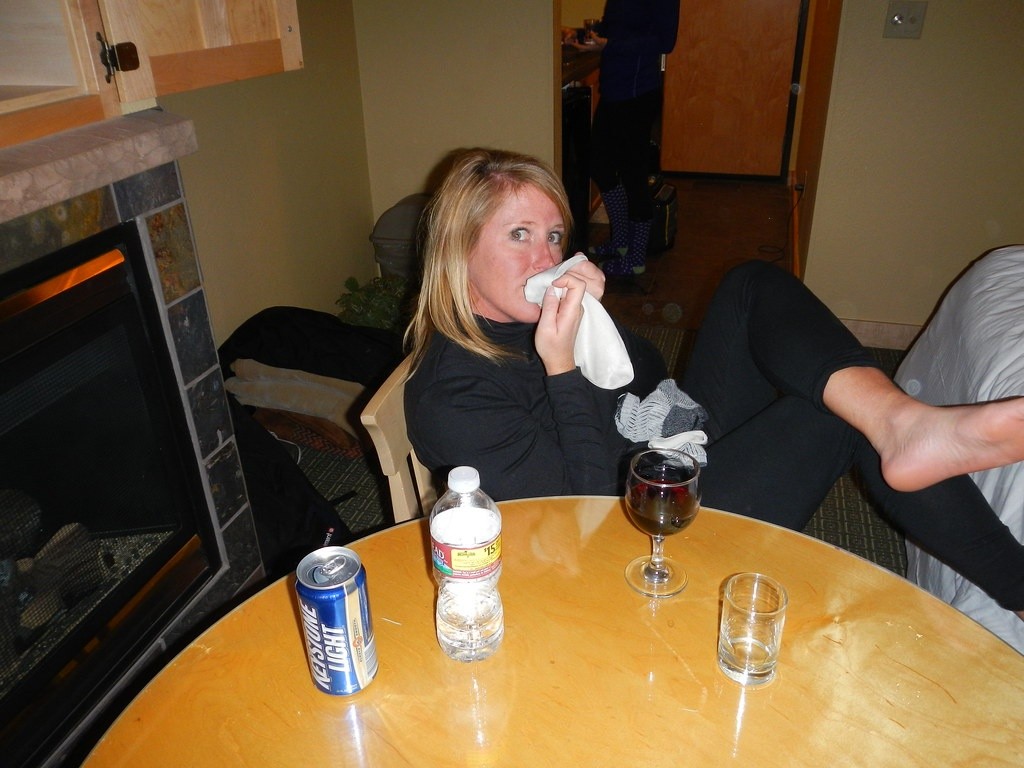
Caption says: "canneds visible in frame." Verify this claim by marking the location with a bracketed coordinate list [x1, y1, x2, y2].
[294, 546, 379, 696]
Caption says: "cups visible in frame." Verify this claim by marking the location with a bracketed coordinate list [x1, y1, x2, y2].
[717, 571, 789, 691]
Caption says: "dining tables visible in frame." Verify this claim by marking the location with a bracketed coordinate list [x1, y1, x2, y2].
[74, 496, 1024, 768]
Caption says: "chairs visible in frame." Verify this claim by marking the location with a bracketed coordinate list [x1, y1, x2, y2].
[358, 352, 445, 526]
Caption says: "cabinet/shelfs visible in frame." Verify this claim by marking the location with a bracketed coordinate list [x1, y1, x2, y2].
[1, 1, 306, 147]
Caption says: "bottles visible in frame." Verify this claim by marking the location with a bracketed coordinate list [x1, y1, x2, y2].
[429, 465, 504, 663]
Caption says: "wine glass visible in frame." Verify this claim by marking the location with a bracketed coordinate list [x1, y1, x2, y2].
[624, 449, 701, 599]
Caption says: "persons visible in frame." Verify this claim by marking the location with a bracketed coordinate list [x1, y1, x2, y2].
[401, 148, 1024, 626]
[563, 1, 681, 278]
